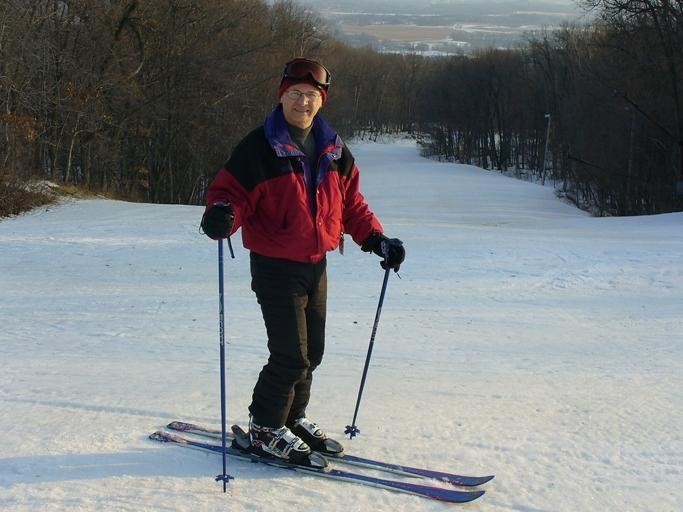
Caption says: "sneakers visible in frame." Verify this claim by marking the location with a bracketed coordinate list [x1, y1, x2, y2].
[249, 417, 326, 464]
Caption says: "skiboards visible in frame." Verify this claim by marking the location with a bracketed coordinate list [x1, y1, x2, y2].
[150, 420, 494, 503]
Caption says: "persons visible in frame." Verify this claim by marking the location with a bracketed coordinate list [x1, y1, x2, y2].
[200, 58, 406, 465]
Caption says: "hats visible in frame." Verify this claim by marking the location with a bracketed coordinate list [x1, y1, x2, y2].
[279, 56, 332, 106]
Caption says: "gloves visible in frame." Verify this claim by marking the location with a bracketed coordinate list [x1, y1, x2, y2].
[201, 204, 235, 240]
[361, 232, 405, 272]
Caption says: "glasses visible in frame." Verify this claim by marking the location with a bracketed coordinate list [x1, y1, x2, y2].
[284, 90, 324, 99]
